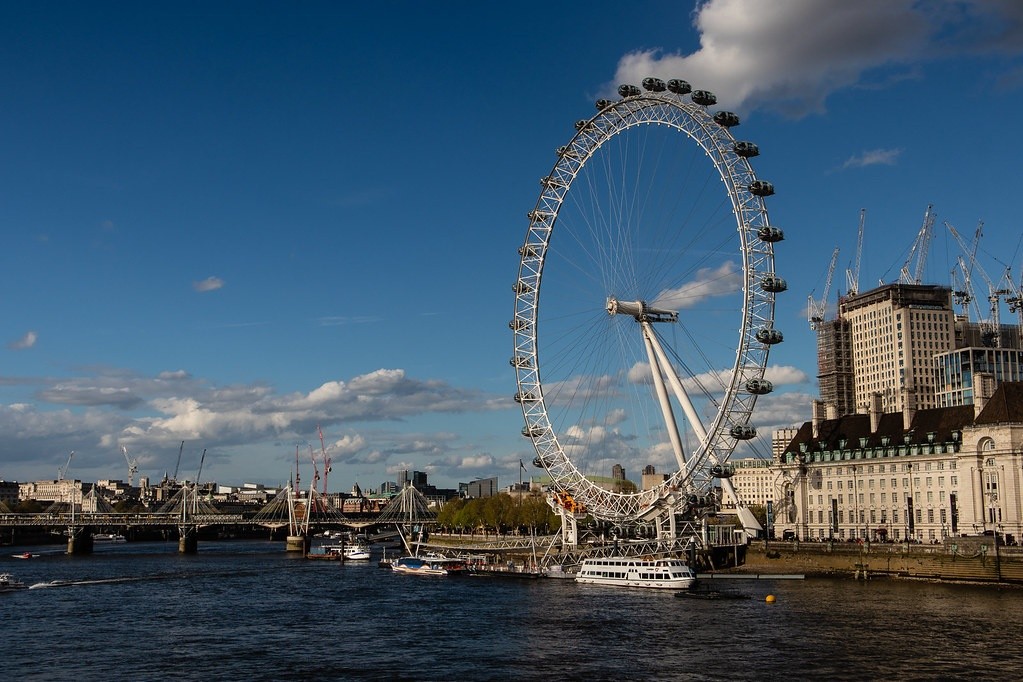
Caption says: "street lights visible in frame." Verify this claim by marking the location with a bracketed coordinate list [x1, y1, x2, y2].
[907, 462, 914, 499]
[853, 464, 860, 544]
[979, 467, 988, 532]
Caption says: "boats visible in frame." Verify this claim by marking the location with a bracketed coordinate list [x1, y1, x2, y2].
[27, 579, 74, 591]
[321, 532, 372, 561]
[11, 553, 40, 559]
[378, 545, 400, 569]
[390, 522, 469, 578]
[0, 573, 30, 589]
[575, 535, 698, 592]
[92, 532, 127, 546]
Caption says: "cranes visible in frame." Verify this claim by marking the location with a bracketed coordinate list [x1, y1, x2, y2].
[120, 444, 140, 487]
[806, 203, 1023, 347]
[56, 450, 75, 482]
[163, 439, 186, 489]
[290, 426, 333, 521]
[187, 449, 218, 495]
